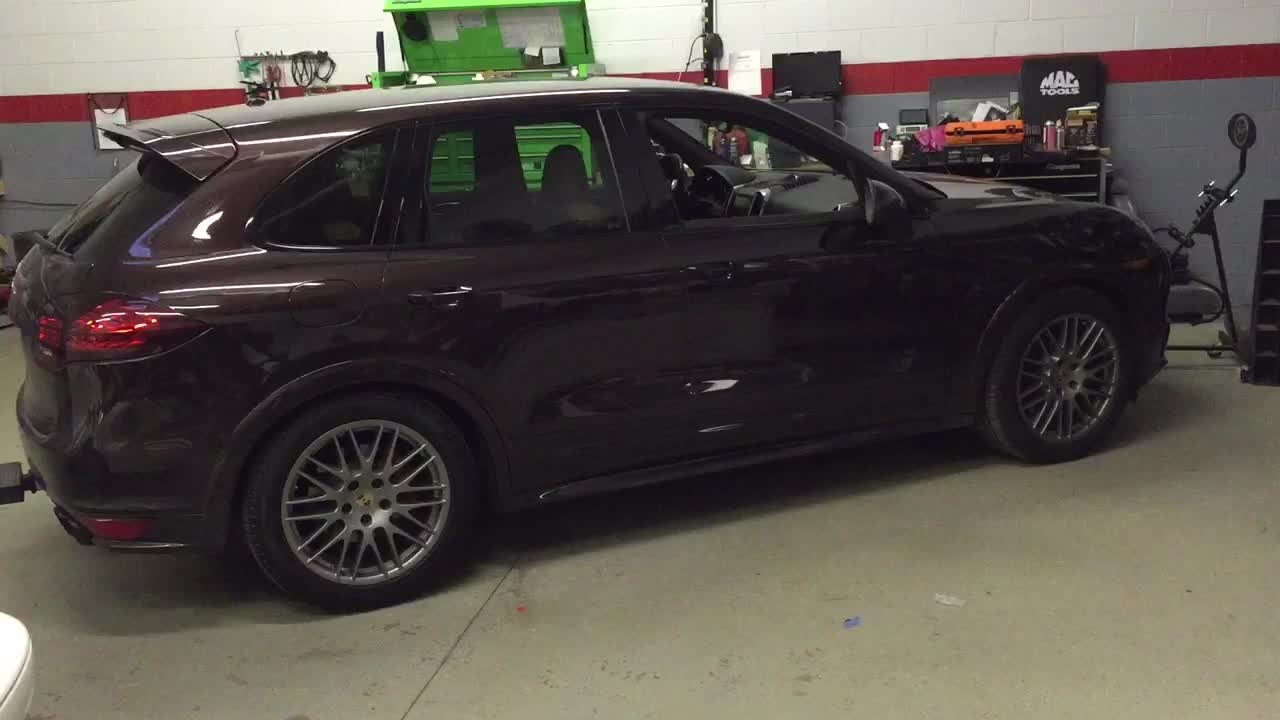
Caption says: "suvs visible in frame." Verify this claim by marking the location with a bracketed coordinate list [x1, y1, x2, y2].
[8, 74, 1175, 618]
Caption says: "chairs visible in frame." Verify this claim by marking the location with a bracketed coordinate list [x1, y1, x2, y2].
[536, 144, 591, 208]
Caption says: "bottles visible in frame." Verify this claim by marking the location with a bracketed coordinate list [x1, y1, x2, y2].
[1055, 119, 1065, 153]
[1042, 119, 1056, 152]
[720, 137, 737, 163]
[891, 141, 904, 161]
